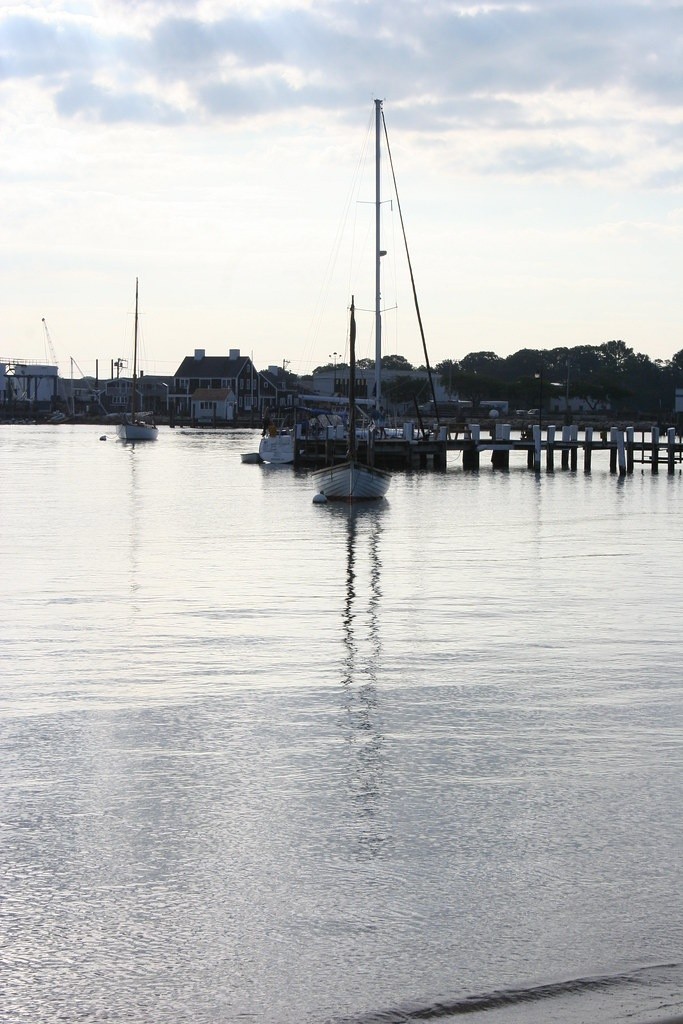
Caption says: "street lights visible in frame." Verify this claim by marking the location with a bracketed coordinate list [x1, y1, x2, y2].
[535, 368, 543, 427]
[328, 352, 342, 396]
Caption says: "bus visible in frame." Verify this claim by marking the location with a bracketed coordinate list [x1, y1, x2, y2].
[418, 400, 508, 418]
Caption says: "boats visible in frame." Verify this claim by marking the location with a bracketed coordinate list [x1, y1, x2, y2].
[259, 428, 305, 464]
[52, 412, 66, 421]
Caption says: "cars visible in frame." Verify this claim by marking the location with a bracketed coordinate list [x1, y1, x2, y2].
[529, 409, 540, 416]
[516, 410, 528, 416]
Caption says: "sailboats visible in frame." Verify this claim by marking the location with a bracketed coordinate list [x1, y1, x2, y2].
[297, 98, 447, 470]
[312, 295, 393, 502]
[116, 276, 158, 442]
